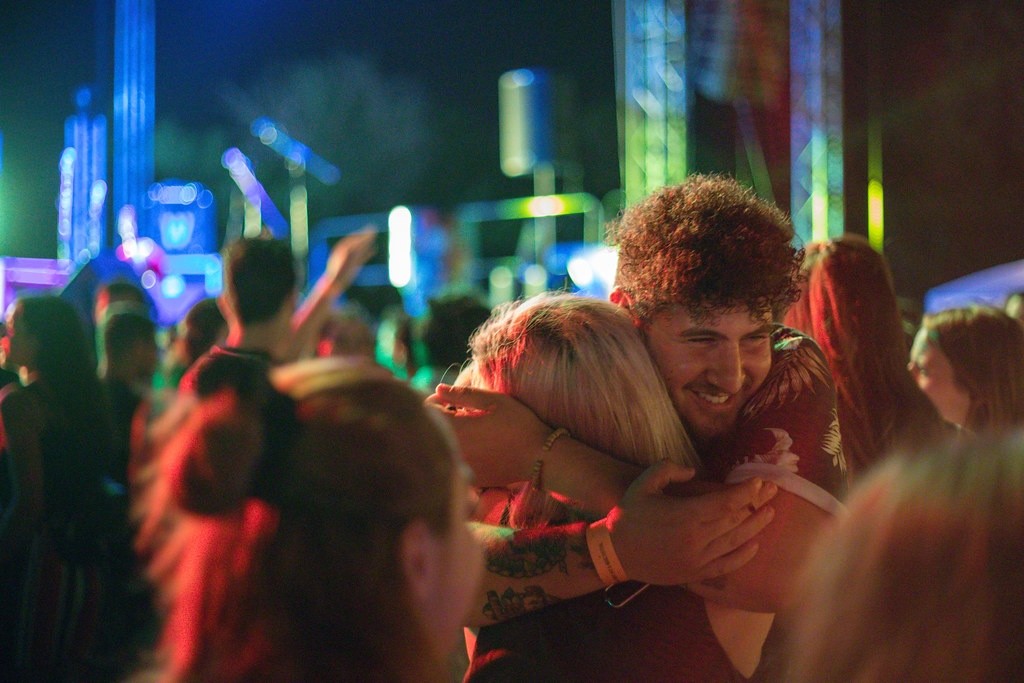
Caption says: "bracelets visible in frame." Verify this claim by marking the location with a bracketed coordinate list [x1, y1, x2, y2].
[533, 427, 572, 491]
[586, 517, 631, 586]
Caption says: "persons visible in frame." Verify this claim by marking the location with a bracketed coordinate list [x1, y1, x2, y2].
[152, 345, 486, 683]
[0, 170, 1024, 683]
